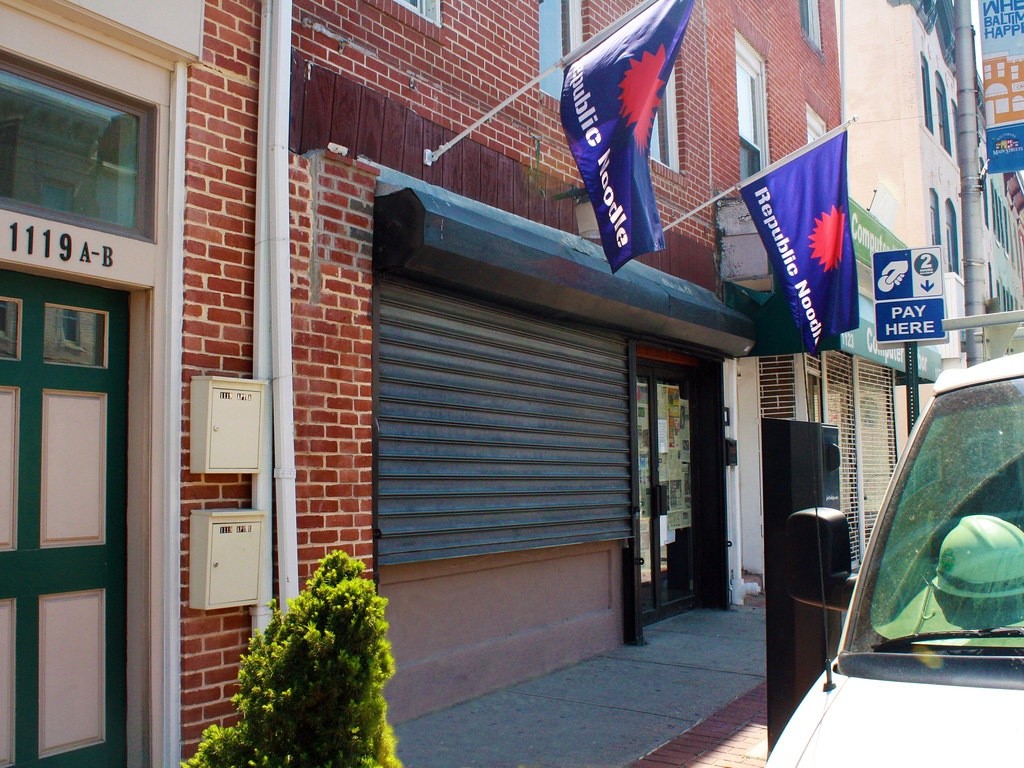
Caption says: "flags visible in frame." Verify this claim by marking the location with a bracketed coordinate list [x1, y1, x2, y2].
[559, 0, 694, 275]
[739, 130, 858, 354]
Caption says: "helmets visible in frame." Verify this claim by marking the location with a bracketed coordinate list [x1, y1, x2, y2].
[931, 514, 1024, 599]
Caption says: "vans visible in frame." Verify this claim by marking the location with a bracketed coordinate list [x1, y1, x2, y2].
[765, 351, 1024, 766]
